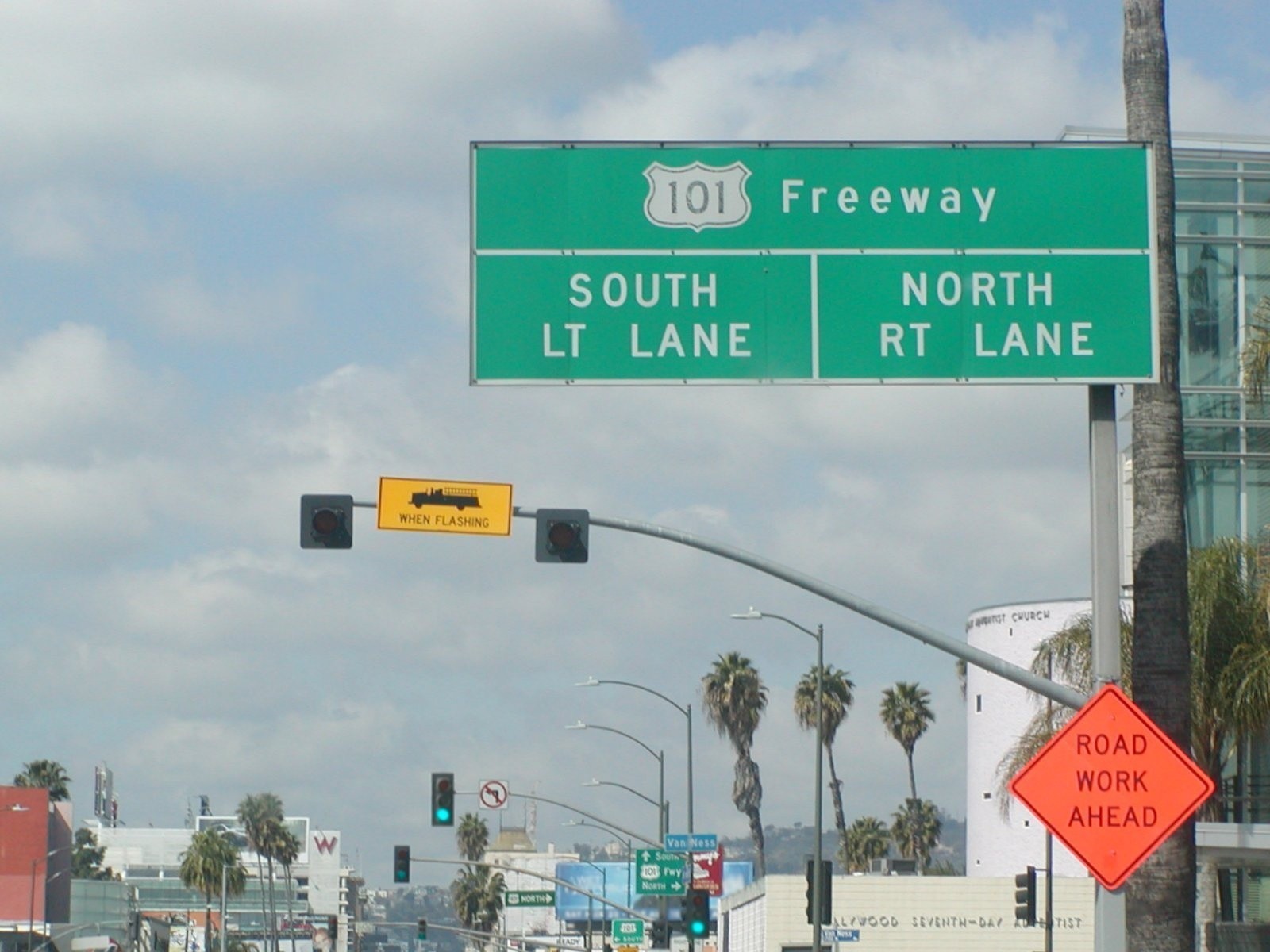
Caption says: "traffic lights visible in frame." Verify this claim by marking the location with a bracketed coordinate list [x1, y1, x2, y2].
[651, 919, 668, 949]
[299, 492, 355, 551]
[534, 507, 590, 564]
[393, 844, 411, 884]
[429, 770, 456, 829]
[684, 889, 710, 941]
[1013, 865, 1038, 928]
[415, 916, 429, 943]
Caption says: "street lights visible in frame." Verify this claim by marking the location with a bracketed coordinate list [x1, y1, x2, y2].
[558, 673, 697, 952]
[729, 605, 825, 951]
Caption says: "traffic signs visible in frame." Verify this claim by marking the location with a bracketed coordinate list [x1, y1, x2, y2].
[610, 918, 646, 945]
[505, 890, 557, 908]
[635, 848, 688, 897]
